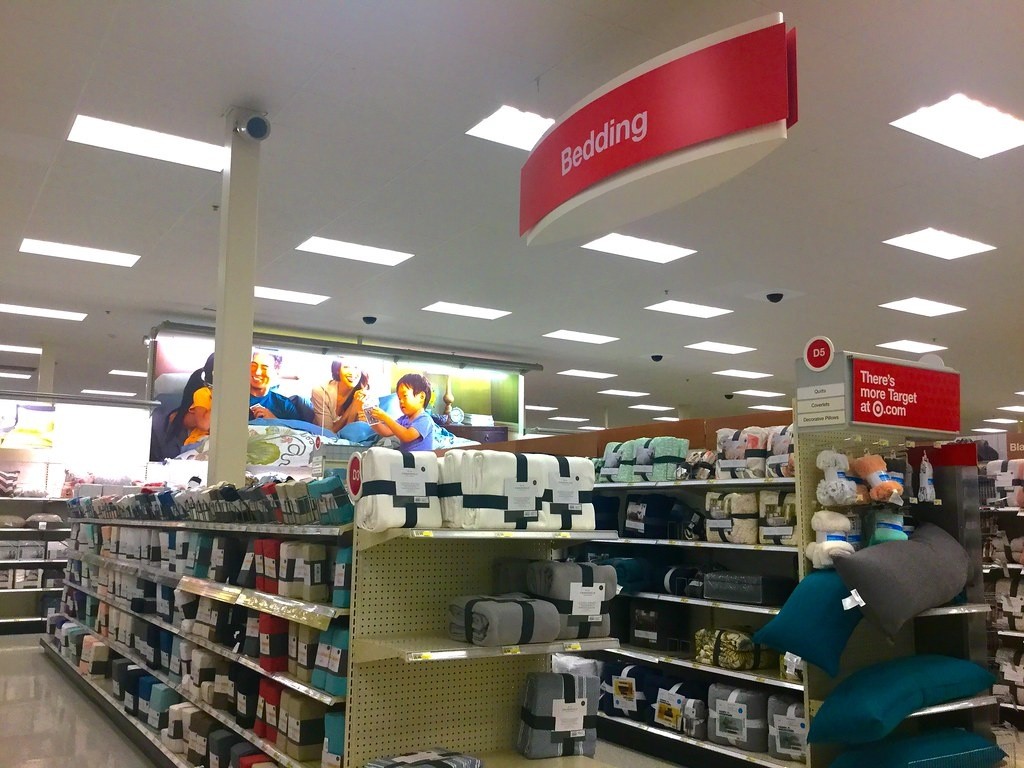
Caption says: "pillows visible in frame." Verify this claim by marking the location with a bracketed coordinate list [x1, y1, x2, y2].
[830, 522, 982, 644]
[805, 653, 993, 746]
[824, 726, 1009, 768]
[752, 571, 858, 677]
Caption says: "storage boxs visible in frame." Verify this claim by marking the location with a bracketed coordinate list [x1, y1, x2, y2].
[703, 572, 777, 605]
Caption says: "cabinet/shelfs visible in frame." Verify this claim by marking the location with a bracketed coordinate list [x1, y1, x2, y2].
[0, 425, 1024, 768]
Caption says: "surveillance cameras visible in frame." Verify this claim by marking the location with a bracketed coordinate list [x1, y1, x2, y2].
[651, 355, 663, 361]
[725, 395, 733, 399]
[363, 317, 376, 324]
[767, 293, 783, 303]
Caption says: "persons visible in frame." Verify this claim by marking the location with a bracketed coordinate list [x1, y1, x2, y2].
[165, 350, 434, 450]
